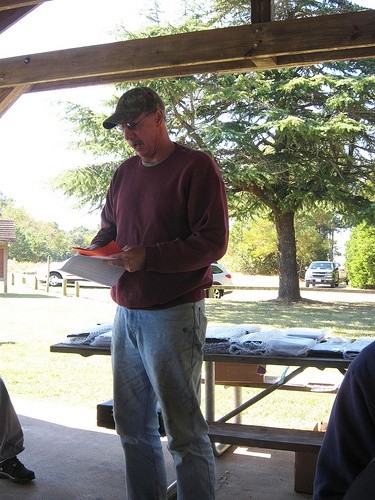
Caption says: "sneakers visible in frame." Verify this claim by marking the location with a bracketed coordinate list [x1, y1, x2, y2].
[0, 456, 36, 484]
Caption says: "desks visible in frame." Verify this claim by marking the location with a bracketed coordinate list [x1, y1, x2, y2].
[51, 343, 354, 499]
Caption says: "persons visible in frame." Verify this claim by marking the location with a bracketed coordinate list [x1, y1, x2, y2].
[0, 378, 35, 482]
[312, 340, 375, 500]
[81, 86, 229, 500]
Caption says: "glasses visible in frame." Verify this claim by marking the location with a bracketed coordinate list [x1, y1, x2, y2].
[117, 111, 152, 130]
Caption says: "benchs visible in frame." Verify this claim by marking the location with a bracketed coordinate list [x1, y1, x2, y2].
[97, 375, 339, 453]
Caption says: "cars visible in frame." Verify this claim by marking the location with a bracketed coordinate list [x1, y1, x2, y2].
[303, 260, 348, 288]
[35, 256, 93, 288]
[204, 261, 236, 300]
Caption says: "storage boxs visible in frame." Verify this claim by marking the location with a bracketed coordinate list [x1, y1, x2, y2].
[293, 420, 330, 493]
[214, 362, 268, 383]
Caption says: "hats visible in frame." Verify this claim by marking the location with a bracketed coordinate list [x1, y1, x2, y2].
[103, 87, 164, 130]
